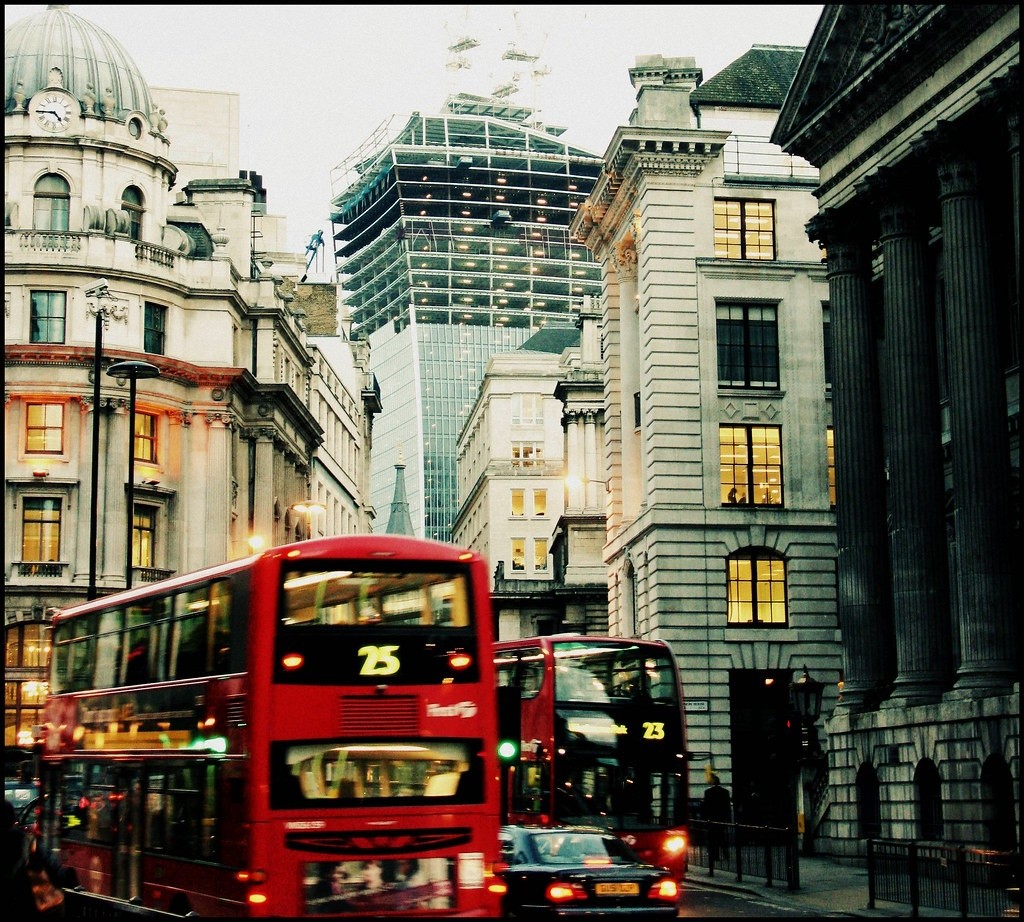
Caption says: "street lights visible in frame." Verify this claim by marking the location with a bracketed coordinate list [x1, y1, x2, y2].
[785, 663, 828, 891]
[104, 360, 162, 591]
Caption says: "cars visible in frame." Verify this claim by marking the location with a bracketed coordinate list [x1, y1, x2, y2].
[499, 823, 682, 922]
[18, 793, 88, 872]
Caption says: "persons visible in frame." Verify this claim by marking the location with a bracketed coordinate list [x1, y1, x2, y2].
[698, 774, 731, 877]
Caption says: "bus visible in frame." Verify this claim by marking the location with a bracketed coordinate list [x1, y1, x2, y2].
[492, 630, 690, 886]
[36, 534, 508, 922]
